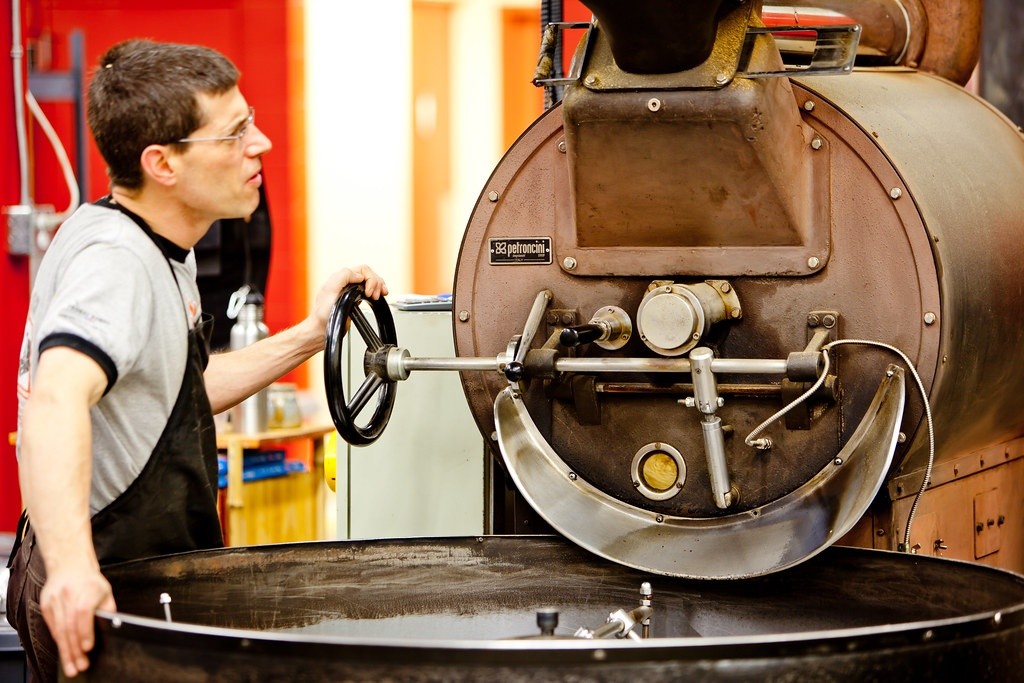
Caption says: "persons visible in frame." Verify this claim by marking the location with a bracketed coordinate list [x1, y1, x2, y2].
[6, 38, 388, 683]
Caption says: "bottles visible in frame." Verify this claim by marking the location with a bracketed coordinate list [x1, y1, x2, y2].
[227, 296, 266, 435]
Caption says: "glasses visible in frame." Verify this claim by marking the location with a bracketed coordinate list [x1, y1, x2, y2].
[178, 105, 256, 144]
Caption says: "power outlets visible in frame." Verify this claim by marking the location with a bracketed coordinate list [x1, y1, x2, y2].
[4, 203, 56, 256]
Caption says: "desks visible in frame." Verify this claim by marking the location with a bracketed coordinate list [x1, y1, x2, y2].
[7, 418, 340, 546]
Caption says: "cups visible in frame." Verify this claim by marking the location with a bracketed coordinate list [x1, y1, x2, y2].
[265, 383, 301, 430]
